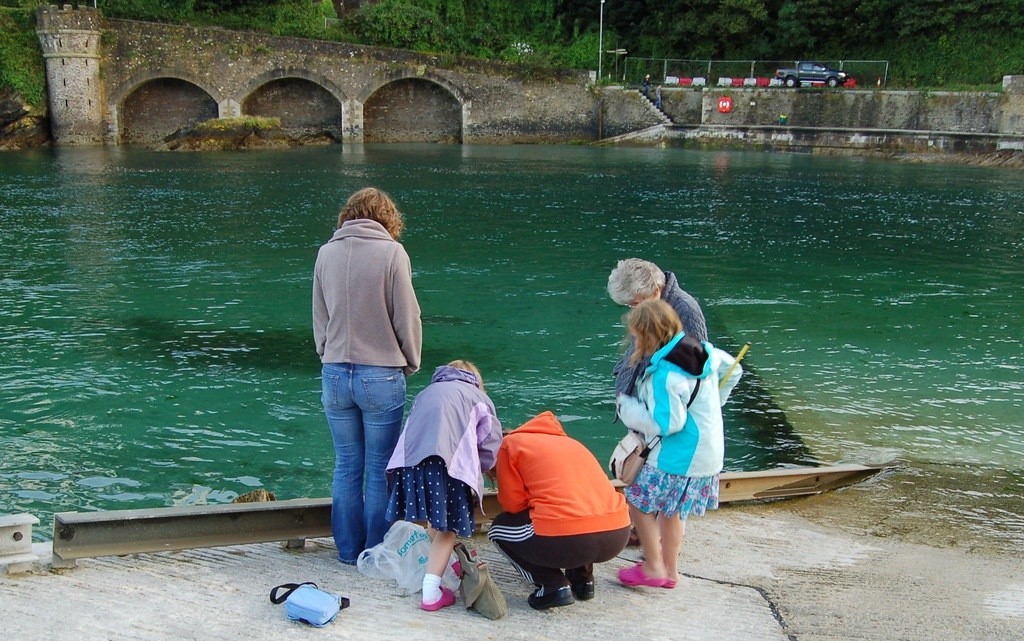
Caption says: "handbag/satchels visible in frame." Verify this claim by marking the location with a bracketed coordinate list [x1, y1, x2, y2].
[452, 542, 506, 620]
[609, 431, 646, 487]
[285, 584, 342, 628]
[357, 520, 460, 596]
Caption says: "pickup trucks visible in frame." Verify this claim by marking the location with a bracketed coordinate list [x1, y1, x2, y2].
[775, 61, 849, 89]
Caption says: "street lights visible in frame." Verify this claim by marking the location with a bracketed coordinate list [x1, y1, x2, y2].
[597, 0, 605, 81]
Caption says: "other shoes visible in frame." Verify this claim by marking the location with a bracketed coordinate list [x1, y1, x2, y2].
[626, 526, 641, 546]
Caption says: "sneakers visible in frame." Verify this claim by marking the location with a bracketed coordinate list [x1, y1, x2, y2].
[565, 569, 594, 600]
[528, 586, 575, 609]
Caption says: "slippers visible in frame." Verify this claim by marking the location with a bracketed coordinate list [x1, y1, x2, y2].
[420, 585, 455, 611]
[662, 579, 678, 589]
[451, 561, 461, 576]
[617, 564, 667, 586]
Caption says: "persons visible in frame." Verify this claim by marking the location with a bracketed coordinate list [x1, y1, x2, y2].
[312, 186, 422, 565]
[608, 258, 708, 548]
[619, 294, 725, 589]
[384, 358, 503, 612]
[485, 410, 631, 611]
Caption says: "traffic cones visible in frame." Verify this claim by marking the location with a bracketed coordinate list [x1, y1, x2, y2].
[876, 77, 881, 89]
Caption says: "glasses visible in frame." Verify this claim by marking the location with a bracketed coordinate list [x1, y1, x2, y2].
[624, 303, 638, 309]
